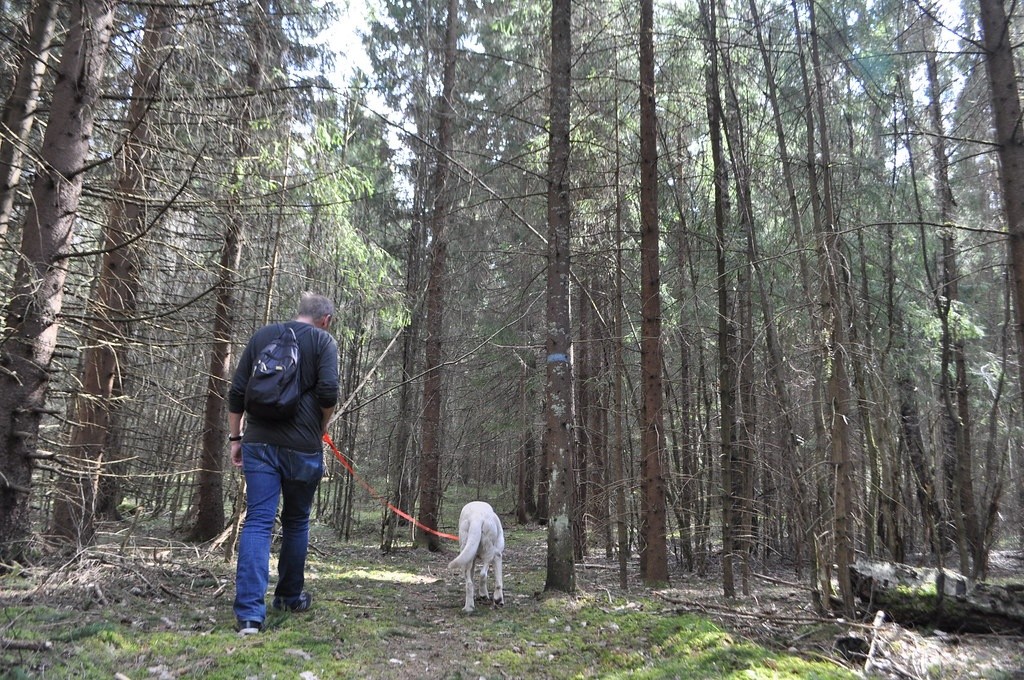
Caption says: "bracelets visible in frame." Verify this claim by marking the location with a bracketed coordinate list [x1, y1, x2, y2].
[229, 436, 241, 441]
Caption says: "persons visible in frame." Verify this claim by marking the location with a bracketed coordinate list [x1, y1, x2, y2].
[228, 292, 339, 635]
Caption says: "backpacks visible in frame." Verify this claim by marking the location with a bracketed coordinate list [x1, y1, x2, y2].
[243, 322, 315, 419]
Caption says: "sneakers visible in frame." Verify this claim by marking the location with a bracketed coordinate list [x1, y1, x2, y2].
[237, 620, 260, 634]
[294, 593, 311, 612]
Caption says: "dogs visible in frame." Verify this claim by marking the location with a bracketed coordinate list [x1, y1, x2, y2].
[448, 501, 506, 613]
